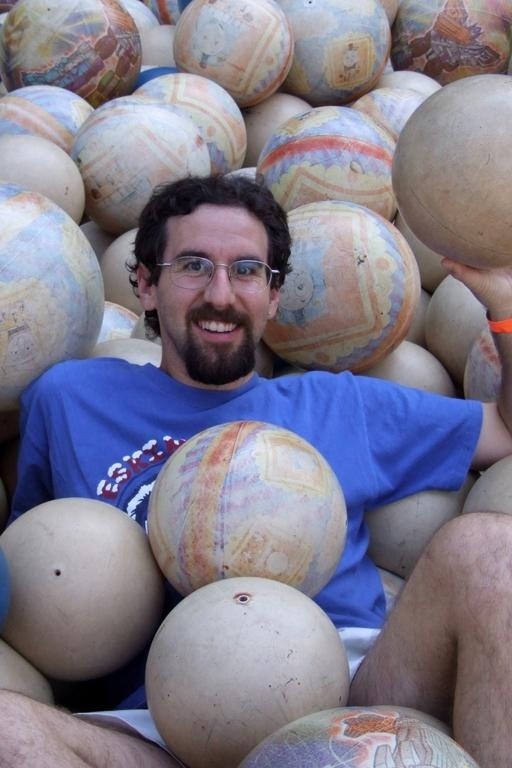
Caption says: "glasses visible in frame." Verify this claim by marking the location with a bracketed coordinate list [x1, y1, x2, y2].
[157, 256, 280, 292]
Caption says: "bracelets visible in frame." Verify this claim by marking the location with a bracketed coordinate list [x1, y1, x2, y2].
[485, 310, 512, 334]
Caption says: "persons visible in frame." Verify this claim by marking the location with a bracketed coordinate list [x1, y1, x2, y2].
[0, 172, 512, 768]
